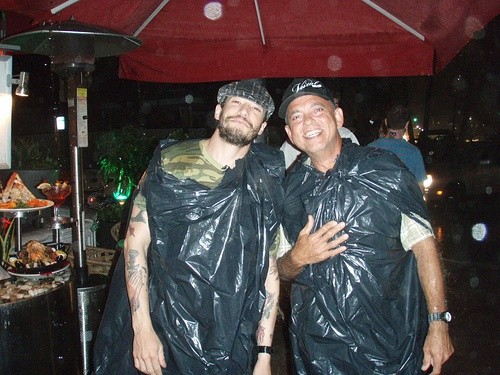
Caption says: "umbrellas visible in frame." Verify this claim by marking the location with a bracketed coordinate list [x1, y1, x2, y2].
[0, 0, 500, 82]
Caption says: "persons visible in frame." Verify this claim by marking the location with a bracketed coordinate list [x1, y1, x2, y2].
[275, 78, 454, 375]
[92, 81, 285, 375]
[368, 104, 427, 186]
[280, 93, 360, 170]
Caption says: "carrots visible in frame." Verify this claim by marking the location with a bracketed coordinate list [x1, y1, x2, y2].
[28, 198, 48, 207]
[0, 202, 14, 209]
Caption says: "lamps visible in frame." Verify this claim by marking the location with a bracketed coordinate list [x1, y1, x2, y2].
[6, 71, 31, 97]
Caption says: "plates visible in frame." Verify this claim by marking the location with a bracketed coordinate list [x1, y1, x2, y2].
[8, 260, 74, 278]
[0, 198, 55, 212]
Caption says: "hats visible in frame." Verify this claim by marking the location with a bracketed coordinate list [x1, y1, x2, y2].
[387, 103, 410, 129]
[217, 78, 275, 122]
[278, 78, 336, 119]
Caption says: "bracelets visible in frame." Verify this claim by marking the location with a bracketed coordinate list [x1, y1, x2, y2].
[256, 346, 272, 355]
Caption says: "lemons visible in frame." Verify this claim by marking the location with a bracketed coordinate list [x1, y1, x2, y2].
[37, 183, 51, 189]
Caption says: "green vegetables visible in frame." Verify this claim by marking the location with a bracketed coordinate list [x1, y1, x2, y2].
[13, 200, 33, 208]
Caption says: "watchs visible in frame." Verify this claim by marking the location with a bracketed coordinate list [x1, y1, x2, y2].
[428, 311, 452, 323]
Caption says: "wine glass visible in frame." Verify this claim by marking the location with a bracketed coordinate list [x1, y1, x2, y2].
[43, 185, 72, 219]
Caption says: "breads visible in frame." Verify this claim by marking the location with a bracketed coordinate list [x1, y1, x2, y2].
[2, 172, 36, 203]
